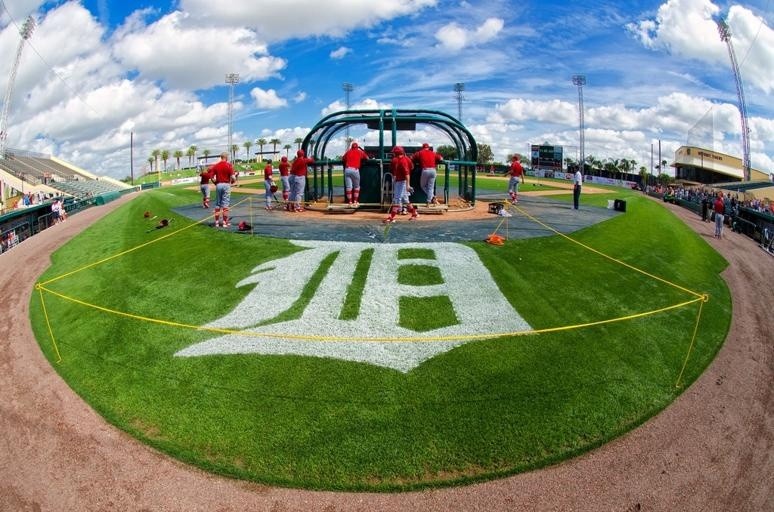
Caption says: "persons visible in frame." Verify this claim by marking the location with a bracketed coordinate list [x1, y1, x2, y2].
[503, 156, 525, 204]
[263, 141, 444, 224]
[209, 152, 236, 227]
[199, 169, 212, 208]
[571, 166, 582, 209]
[631, 184, 774, 253]
[0, 189, 96, 253]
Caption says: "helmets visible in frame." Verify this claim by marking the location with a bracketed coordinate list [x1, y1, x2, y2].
[297, 150, 304, 155]
[352, 143, 357, 147]
[282, 157, 287, 161]
[270, 186, 277, 192]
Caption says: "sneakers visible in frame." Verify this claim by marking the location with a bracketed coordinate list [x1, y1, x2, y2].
[409, 213, 419, 220]
[384, 217, 395, 223]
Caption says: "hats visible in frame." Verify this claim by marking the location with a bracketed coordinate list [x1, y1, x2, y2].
[423, 143, 428, 148]
[392, 146, 401, 152]
